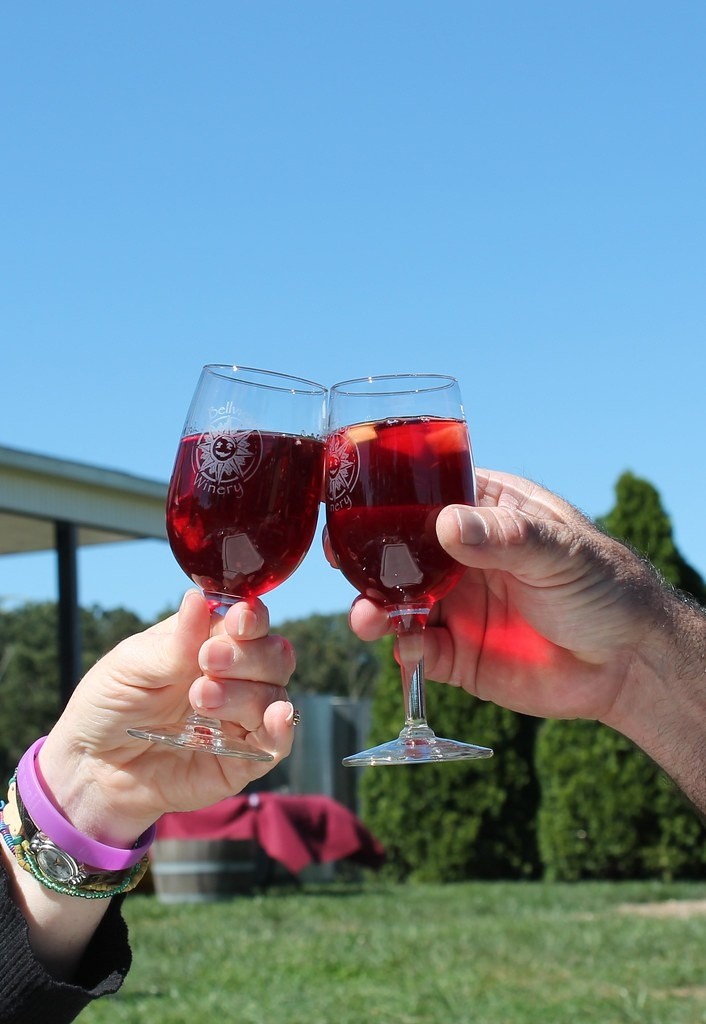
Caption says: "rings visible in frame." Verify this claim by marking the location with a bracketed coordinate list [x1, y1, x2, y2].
[288, 700, 300, 727]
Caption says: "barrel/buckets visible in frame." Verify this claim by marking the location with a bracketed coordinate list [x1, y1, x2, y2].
[149, 833, 262, 903]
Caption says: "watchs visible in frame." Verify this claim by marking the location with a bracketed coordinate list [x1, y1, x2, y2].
[12, 776, 133, 888]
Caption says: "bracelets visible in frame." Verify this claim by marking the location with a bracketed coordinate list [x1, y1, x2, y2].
[0, 766, 150, 900]
[15, 734, 157, 871]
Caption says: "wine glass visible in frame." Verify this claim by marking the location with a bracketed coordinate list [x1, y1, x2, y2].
[126, 363, 328, 762]
[324, 374, 495, 767]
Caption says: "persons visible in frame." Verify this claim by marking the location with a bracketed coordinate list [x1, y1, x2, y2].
[319, 463, 706, 823]
[0, 587, 300, 1024]
